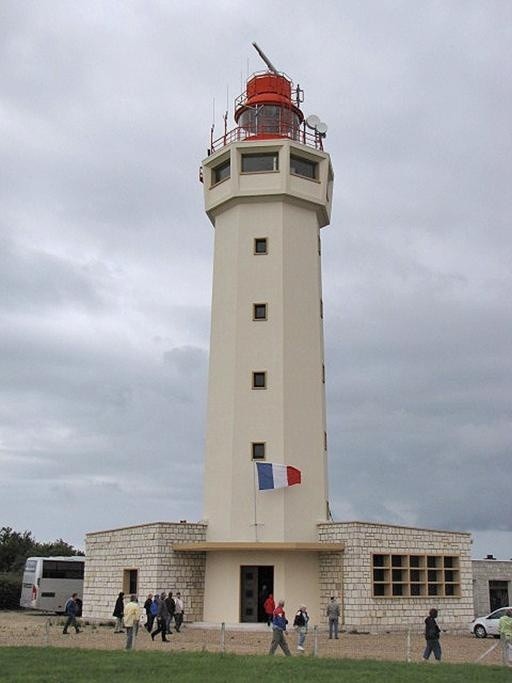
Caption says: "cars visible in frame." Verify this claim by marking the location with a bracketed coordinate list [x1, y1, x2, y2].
[469, 606, 512, 639]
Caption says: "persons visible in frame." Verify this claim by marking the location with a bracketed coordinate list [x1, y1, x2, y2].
[499, 608, 512, 667]
[263, 594, 275, 627]
[325, 595, 340, 639]
[62, 592, 84, 634]
[293, 606, 309, 651]
[269, 600, 292, 655]
[112, 592, 184, 650]
[259, 585, 269, 609]
[422, 608, 442, 662]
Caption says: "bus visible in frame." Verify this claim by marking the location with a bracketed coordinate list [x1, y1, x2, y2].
[18, 554, 84, 617]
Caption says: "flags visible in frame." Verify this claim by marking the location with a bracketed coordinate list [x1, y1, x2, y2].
[256, 461, 301, 490]
